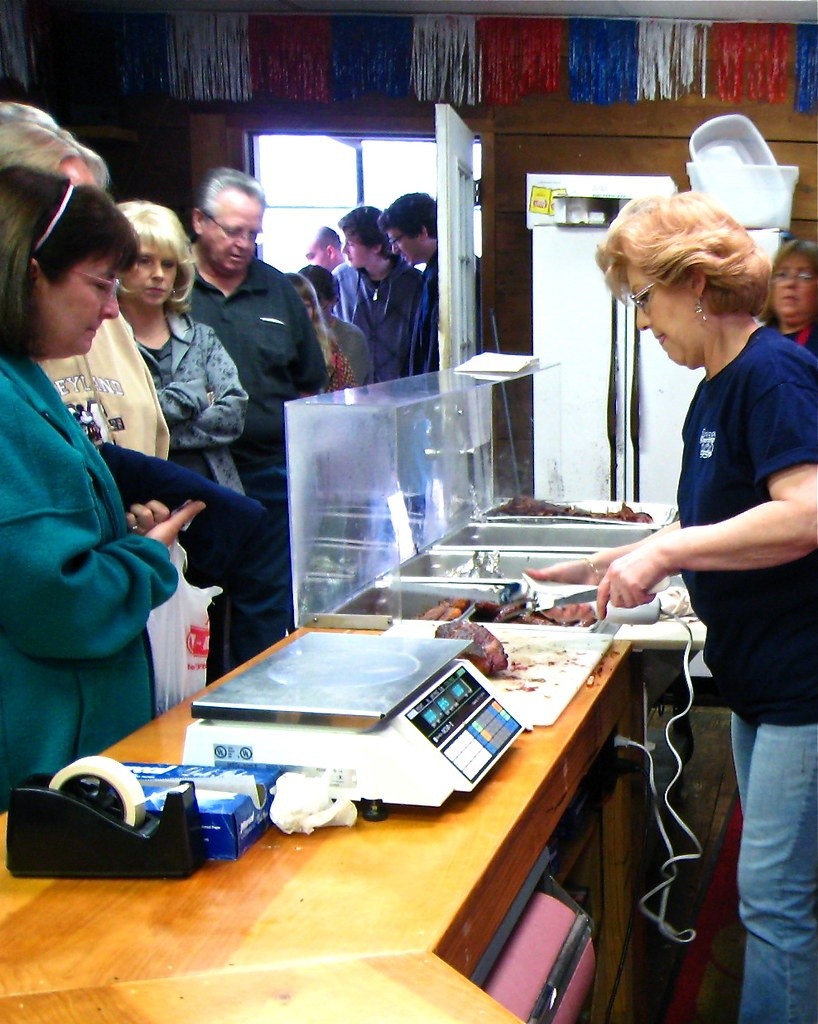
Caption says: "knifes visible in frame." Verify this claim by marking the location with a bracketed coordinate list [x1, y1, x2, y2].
[553, 575, 671, 605]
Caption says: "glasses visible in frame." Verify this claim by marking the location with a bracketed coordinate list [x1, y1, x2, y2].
[629, 281, 661, 314]
[774, 269, 818, 284]
[389, 231, 406, 247]
[67, 265, 120, 303]
[199, 210, 262, 246]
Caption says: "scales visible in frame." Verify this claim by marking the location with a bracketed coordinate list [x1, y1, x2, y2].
[181, 631, 532, 824]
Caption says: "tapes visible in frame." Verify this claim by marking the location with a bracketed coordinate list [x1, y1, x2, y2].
[47, 755, 146, 828]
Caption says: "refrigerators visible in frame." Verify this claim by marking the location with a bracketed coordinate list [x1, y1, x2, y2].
[534, 227, 782, 586]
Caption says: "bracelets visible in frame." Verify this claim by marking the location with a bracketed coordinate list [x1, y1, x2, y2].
[584, 557, 601, 585]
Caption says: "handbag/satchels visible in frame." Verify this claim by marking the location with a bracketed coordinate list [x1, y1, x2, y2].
[144, 534, 225, 718]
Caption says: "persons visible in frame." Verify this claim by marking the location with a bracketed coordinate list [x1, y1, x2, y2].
[300, 224, 376, 341]
[339, 206, 424, 386]
[1, 101, 172, 463]
[298, 264, 373, 388]
[0, 167, 207, 815]
[757, 239, 818, 359]
[283, 273, 359, 399]
[524, 191, 818, 1024]
[114, 199, 248, 497]
[378, 192, 440, 376]
[188, 168, 331, 558]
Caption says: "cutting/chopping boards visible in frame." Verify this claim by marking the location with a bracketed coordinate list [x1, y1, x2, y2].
[380, 621, 614, 726]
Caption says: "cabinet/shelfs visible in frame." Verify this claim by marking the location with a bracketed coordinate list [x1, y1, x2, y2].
[0, 627, 670, 1024]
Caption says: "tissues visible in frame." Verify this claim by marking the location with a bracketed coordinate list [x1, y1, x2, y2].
[82, 759, 287, 861]
